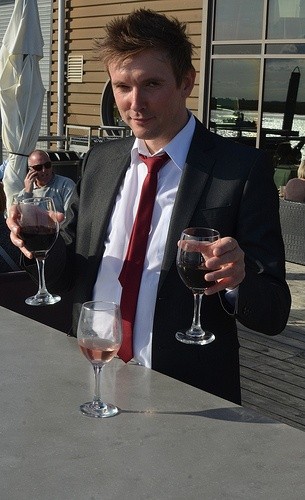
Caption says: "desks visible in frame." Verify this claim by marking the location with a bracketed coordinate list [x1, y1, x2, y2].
[215, 122, 299, 145]
[275, 163, 298, 187]
[0, 305, 305, 499]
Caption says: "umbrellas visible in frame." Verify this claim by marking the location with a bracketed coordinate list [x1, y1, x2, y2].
[0, 0, 47, 209]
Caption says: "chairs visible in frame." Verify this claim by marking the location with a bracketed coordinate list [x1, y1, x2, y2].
[279, 197, 305, 268]
[0, 254, 89, 333]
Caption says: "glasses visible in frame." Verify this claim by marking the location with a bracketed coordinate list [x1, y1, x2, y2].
[29, 161, 51, 171]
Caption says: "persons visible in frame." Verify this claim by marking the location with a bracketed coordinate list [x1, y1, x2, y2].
[12, 150, 76, 216]
[284, 160, 305, 203]
[7, 10, 292, 407]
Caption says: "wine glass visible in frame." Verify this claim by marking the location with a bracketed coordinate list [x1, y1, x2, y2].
[76, 300, 122, 417]
[175, 227, 221, 345]
[18, 197, 61, 306]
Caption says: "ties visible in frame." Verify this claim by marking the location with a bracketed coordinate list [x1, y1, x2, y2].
[113, 152, 172, 364]
